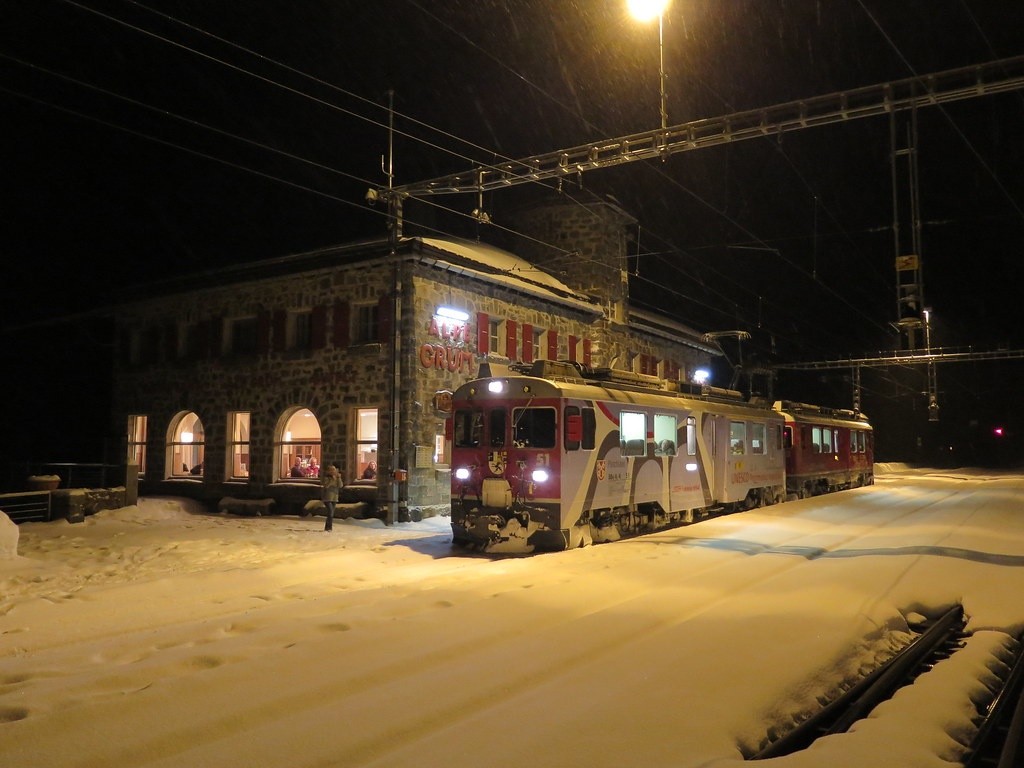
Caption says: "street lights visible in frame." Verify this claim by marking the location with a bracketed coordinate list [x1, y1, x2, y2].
[923, 310, 931, 356]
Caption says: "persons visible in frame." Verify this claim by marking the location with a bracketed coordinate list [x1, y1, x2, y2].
[304, 456, 320, 477]
[183, 461, 203, 476]
[363, 461, 376, 479]
[291, 457, 306, 476]
[323, 465, 343, 532]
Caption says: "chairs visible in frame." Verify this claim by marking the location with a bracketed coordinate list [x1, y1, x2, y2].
[624, 439, 644, 455]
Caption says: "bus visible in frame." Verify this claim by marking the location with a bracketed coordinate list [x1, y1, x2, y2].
[445, 357, 876, 555]
